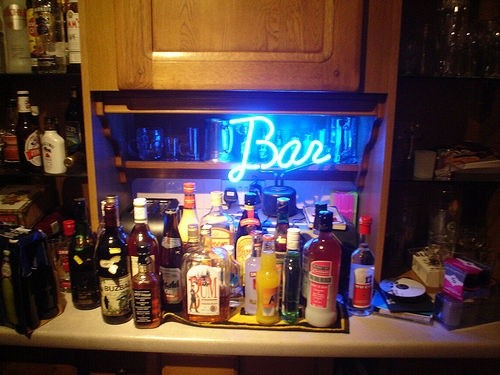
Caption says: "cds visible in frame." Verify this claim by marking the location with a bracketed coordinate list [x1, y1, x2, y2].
[379, 277, 426, 297]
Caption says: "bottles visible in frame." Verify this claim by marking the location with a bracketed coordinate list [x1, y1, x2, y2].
[0, 85, 85, 174]
[0, 181, 376, 331]
[0, 0, 81, 74]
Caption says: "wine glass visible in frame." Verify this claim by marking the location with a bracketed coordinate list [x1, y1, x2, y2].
[398, 5, 500, 77]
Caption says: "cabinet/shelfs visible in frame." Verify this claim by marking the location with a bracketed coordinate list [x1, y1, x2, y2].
[394, 47, 500, 266]
[92, 100, 381, 189]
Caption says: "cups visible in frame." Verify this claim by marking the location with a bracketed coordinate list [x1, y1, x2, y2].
[134, 119, 357, 165]
